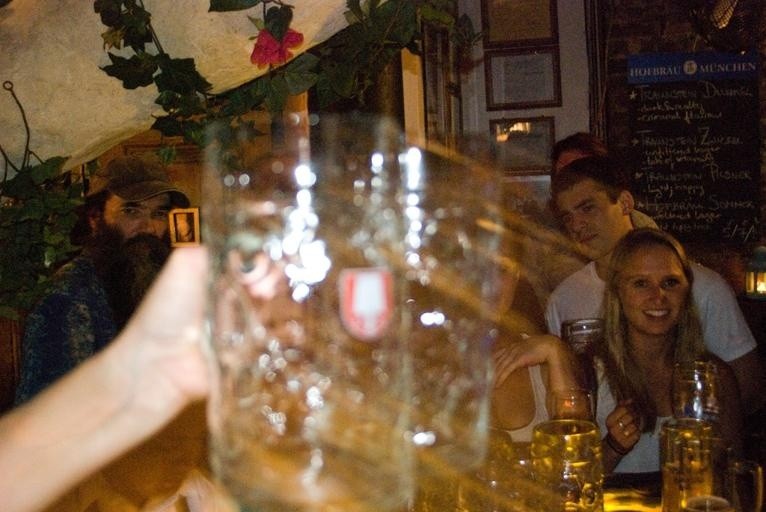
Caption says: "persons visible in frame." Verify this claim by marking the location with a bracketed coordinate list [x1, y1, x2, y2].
[14, 155, 191, 406]
[472, 230, 590, 442]
[574, 228, 747, 477]
[546, 132, 657, 230]
[544, 156, 765, 414]
[1, 245, 306, 512]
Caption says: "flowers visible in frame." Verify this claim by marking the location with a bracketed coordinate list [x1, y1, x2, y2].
[207, 0, 309, 68]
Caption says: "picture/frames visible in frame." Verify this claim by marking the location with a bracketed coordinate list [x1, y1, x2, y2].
[584, 1, 766, 291]
[489, 118, 558, 176]
[483, 48, 561, 109]
[481, 0, 561, 45]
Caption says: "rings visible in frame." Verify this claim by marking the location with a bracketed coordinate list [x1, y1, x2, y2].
[622, 429, 630, 437]
[619, 420, 624, 429]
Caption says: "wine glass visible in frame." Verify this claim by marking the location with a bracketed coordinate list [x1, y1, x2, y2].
[544, 390, 597, 421]
[671, 361, 726, 423]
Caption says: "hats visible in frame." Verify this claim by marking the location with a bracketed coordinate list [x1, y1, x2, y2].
[85, 154, 191, 209]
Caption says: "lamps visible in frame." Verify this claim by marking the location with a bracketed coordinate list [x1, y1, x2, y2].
[740, 245, 766, 297]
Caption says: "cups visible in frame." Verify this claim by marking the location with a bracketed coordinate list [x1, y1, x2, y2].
[659, 419, 715, 512]
[678, 436, 732, 511]
[531, 421, 604, 512]
[730, 459, 763, 511]
[492, 434, 558, 512]
[564, 317, 606, 359]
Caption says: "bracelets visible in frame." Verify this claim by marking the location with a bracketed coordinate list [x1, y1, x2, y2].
[605, 438, 625, 456]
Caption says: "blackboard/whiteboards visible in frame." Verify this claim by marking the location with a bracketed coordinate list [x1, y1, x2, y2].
[584, 0, 765, 248]
